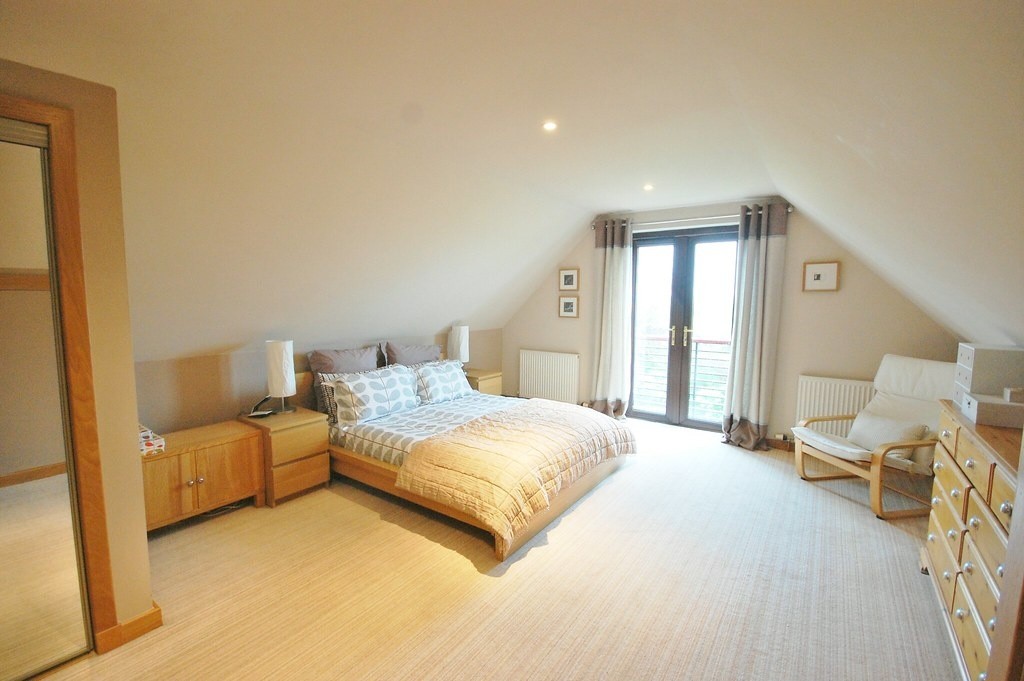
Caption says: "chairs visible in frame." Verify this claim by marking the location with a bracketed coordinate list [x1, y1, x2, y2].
[790, 353, 956, 519]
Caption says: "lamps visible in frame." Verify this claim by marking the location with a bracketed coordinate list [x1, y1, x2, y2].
[452, 326, 470, 368]
[265, 338, 297, 415]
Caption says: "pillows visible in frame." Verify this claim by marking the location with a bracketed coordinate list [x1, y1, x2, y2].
[306, 343, 476, 428]
[849, 412, 936, 468]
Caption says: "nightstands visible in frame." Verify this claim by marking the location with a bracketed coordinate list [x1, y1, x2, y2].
[462, 366, 503, 395]
[238, 405, 330, 507]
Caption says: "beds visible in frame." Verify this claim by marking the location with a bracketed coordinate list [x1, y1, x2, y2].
[289, 353, 636, 563]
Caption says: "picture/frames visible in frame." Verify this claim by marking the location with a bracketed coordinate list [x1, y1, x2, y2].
[801, 260, 842, 292]
[558, 267, 580, 291]
[559, 295, 579, 317]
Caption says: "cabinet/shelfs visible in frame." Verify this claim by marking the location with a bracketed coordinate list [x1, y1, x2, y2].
[921, 397, 1022, 681]
[138, 419, 267, 529]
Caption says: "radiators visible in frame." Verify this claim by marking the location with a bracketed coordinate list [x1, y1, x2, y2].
[519, 349, 580, 405]
[797, 374, 877, 438]
